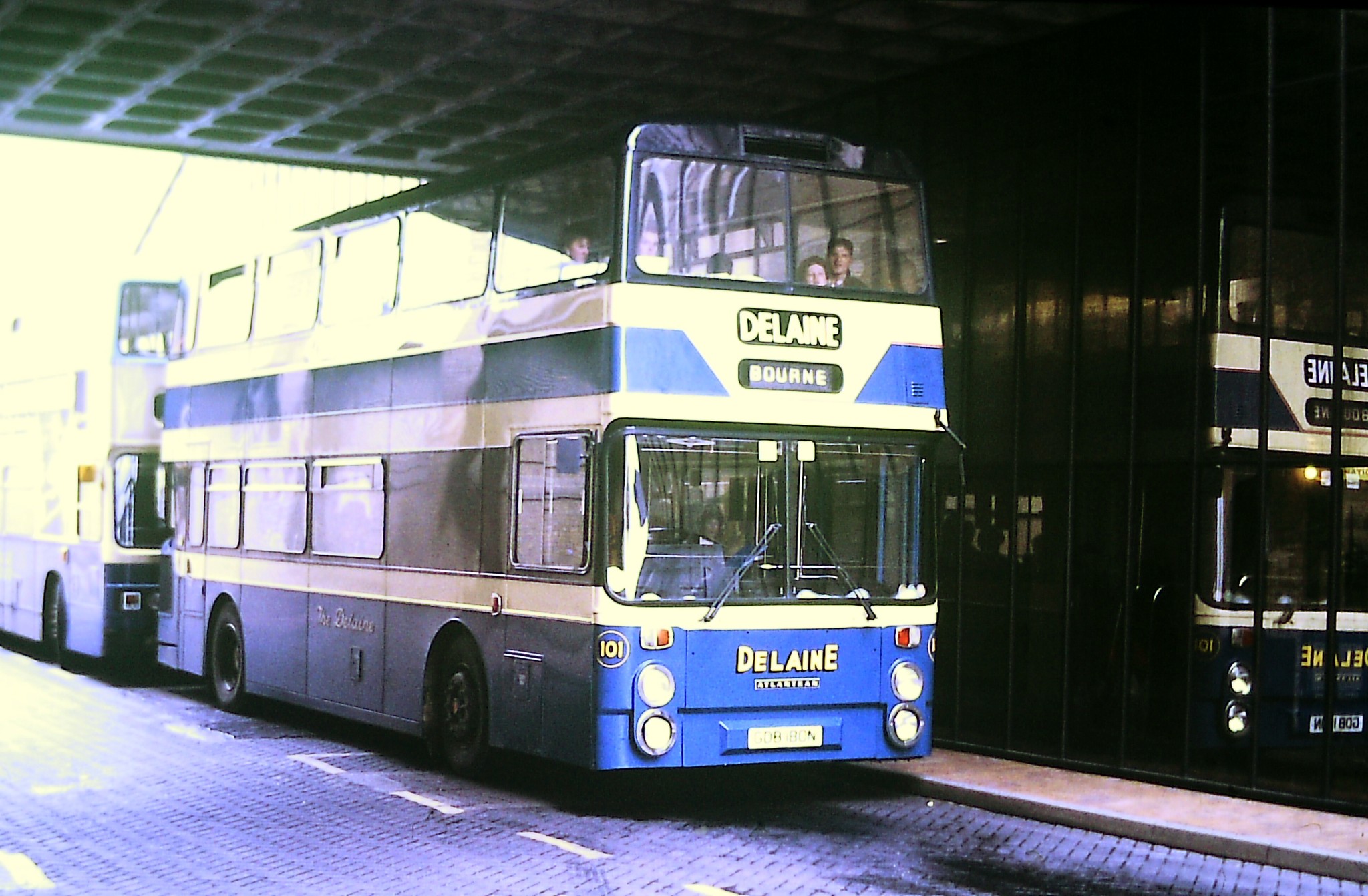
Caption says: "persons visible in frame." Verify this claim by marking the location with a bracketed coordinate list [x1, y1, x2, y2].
[647, 516, 667, 545]
[545, 225, 591, 267]
[1020, 533, 1043, 575]
[824, 237, 869, 289]
[685, 503, 724, 545]
[796, 256, 829, 286]
[707, 252, 732, 274]
[978, 525, 1019, 576]
[939, 513, 978, 577]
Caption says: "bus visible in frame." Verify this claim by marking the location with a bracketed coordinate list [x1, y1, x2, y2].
[7, 281, 186, 669]
[160, 122, 944, 758]
